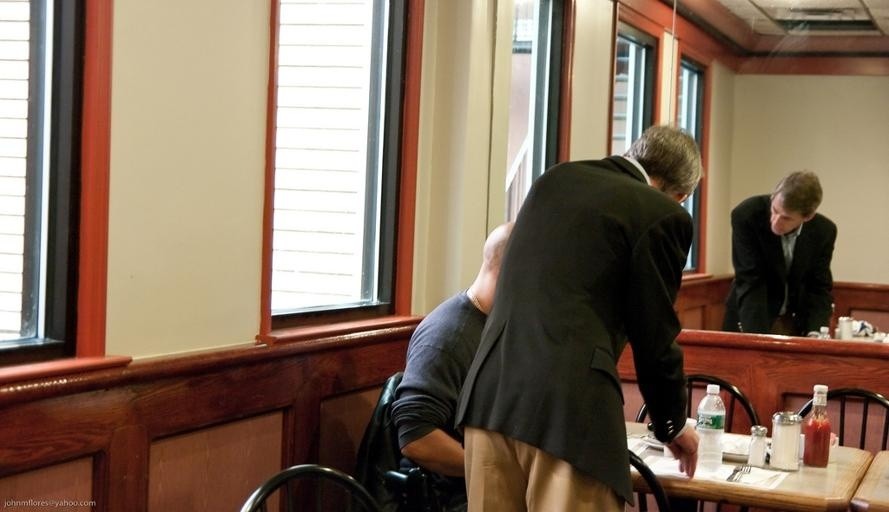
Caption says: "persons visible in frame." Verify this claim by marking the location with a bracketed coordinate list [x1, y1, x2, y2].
[452, 125, 705, 512]
[721, 170, 839, 337]
[391, 219, 518, 512]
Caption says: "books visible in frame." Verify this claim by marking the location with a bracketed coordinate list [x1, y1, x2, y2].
[626, 435, 649, 457]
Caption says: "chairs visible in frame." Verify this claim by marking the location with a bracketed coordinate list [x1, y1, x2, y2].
[798, 388, 888, 451]
[382, 372, 430, 512]
[635, 374, 761, 512]
[240, 464, 380, 512]
[628, 449, 669, 512]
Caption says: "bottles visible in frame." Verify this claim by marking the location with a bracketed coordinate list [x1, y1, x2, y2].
[694, 384, 726, 481]
[801, 384, 832, 468]
[817, 326, 831, 340]
[769, 411, 804, 470]
[747, 425, 768, 468]
